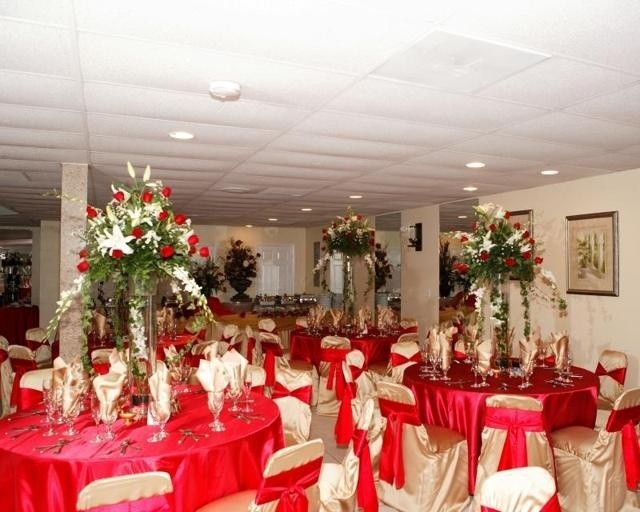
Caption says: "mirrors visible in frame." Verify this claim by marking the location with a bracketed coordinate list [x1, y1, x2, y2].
[373, 208, 402, 332]
[436, 198, 483, 333]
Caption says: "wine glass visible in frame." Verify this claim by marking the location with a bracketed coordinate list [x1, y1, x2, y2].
[416, 325, 573, 386]
[33, 362, 256, 442]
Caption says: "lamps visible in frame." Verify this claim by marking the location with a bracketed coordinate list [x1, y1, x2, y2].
[400, 222, 423, 252]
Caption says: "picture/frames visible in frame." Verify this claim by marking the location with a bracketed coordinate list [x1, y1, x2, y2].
[500, 208, 535, 282]
[564, 209, 621, 298]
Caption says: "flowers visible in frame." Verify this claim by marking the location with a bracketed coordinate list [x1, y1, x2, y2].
[216, 238, 263, 278]
[1, 251, 33, 273]
[440, 236, 458, 299]
[311, 211, 382, 295]
[42, 158, 214, 385]
[444, 203, 570, 356]
[186, 255, 226, 292]
[375, 240, 394, 293]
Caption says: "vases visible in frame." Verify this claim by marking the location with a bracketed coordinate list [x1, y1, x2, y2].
[341, 249, 355, 331]
[229, 280, 252, 302]
[489, 271, 511, 370]
[124, 272, 160, 422]
[6, 272, 21, 297]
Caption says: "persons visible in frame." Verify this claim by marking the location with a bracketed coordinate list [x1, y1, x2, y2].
[439, 256, 453, 298]
[450, 255, 465, 298]
[6, 267, 21, 289]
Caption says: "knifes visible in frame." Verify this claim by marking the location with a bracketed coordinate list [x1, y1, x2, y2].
[32, 438, 67, 455]
[104, 439, 134, 460]
[4, 409, 38, 440]
